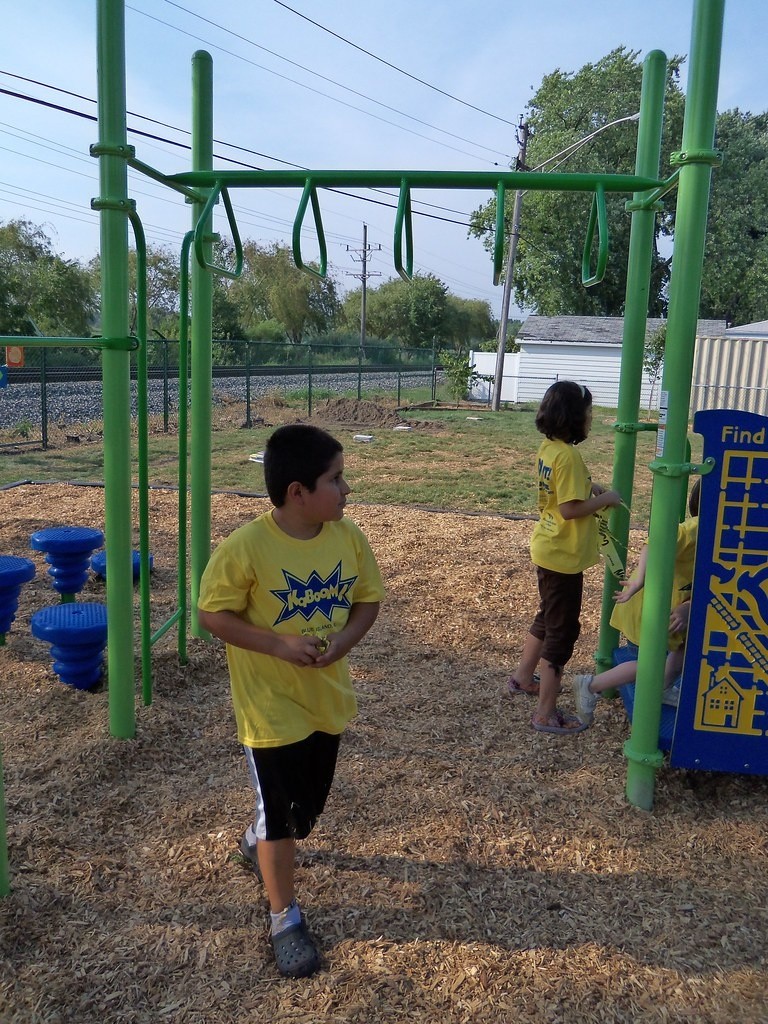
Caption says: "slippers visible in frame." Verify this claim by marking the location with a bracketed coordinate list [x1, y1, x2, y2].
[270, 922, 317, 979]
[240, 834, 264, 881]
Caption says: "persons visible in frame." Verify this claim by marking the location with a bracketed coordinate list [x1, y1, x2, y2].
[506, 382, 623, 737]
[199, 424, 387, 977]
[572, 475, 702, 729]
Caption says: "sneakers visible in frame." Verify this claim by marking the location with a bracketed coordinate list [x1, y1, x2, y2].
[572, 674, 602, 725]
[662, 685, 679, 707]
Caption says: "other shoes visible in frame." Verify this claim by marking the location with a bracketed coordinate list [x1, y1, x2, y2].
[531, 708, 588, 732]
[508, 674, 564, 695]
